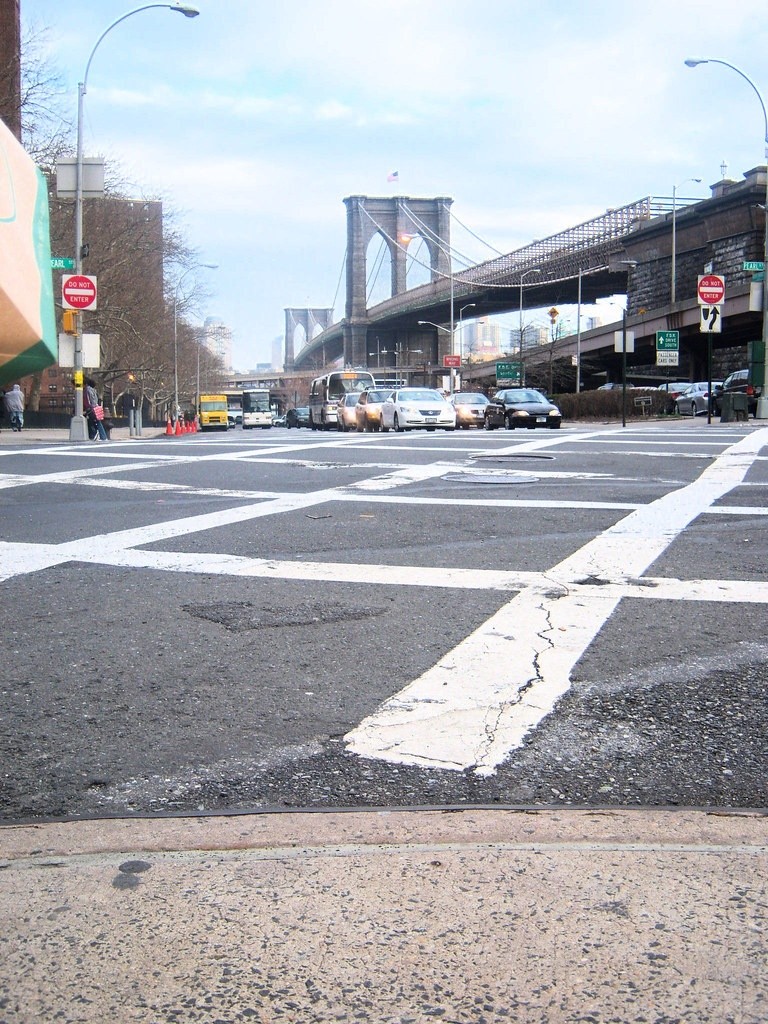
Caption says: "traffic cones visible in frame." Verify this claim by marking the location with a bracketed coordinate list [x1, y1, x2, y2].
[164, 417, 198, 435]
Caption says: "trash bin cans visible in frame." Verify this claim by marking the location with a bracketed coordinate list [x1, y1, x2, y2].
[720, 384, 749, 423]
[184, 409, 194, 427]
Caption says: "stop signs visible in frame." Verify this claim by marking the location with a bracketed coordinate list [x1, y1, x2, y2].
[698, 274, 724, 305]
[62, 274, 97, 311]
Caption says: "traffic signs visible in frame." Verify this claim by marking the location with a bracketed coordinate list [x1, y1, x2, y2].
[657, 330, 679, 351]
[497, 362, 525, 383]
[82, 244, 89, 258]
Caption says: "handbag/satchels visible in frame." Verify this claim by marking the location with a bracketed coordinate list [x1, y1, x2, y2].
[93, 406, 105, 420]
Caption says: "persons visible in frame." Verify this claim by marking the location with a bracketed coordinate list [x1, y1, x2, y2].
[71, 375, 108, 442]
[4, 384, 25, 431]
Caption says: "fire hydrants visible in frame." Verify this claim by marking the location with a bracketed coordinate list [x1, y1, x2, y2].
[102, 407, 114, 439]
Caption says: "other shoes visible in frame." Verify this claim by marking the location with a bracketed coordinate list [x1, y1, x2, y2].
[93, 430, 99, 441]
[97, 439, 108, 442]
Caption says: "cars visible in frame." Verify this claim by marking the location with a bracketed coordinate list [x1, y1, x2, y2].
[445, 392, 491, 430]
[596, 370, 757, 418]
[273, 407, 310, 429]
[484, 389, 563, 430]
[228, 416, 242, 429]
[336, 385, 457, 432]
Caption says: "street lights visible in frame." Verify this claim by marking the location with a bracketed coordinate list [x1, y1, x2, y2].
[519, 269, 540, 390]
[174, 263, 226, 430]
[70, 2, 199, 442]
[670, 178, 703, 304]
[459, 304, 476, 365]
[400, 234, 485, 396]
[577, 260, 638, 393]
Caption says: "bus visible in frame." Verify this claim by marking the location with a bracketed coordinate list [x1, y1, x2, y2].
[309, 370, 377, 430]
[200, 395, 230, 432]
[223, 388, 272, 429]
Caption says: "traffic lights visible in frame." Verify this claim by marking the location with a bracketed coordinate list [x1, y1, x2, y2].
[63, 313, 76, 334]
